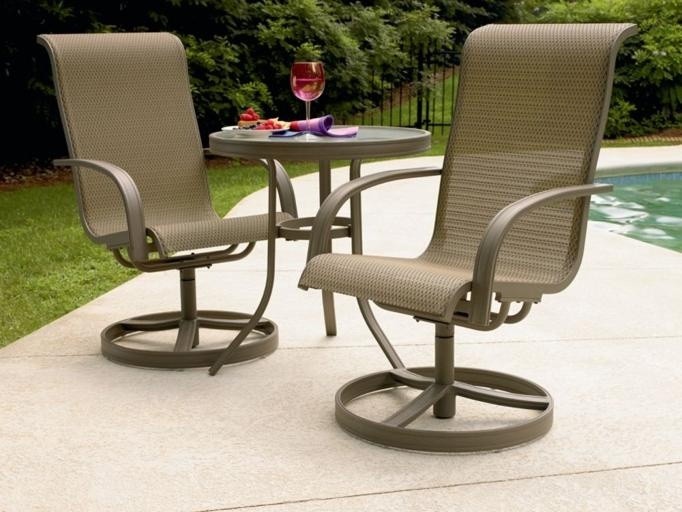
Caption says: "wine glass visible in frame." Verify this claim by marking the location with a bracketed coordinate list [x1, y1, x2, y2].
[288, 60, 327, 141]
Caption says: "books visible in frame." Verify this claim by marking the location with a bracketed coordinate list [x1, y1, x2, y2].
[268, 130, 303, 138]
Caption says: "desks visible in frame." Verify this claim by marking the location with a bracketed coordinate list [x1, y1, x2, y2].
[208, 125, 432, 375]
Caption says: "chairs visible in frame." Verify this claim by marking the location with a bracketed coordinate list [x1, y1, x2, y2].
[297, 21, 640, 454]
[37, 32, 296, 368]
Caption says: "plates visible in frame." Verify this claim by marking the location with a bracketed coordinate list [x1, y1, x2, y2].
[219, 123, 290, 136]
